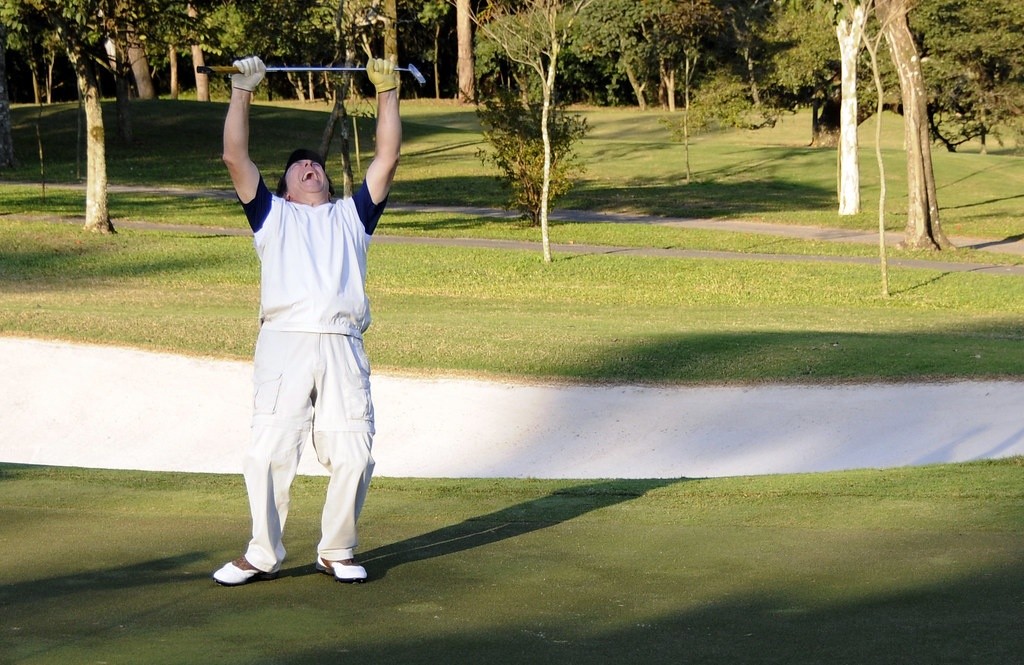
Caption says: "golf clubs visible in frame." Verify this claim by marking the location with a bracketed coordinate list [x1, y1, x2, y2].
[195, 63, 428, 87]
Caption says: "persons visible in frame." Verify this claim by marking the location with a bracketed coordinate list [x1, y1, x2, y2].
[212, 56, 402, 587]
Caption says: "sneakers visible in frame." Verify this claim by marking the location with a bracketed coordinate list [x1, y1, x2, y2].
[213, 556, 267, 586]
[315, 554, 367, 583]
